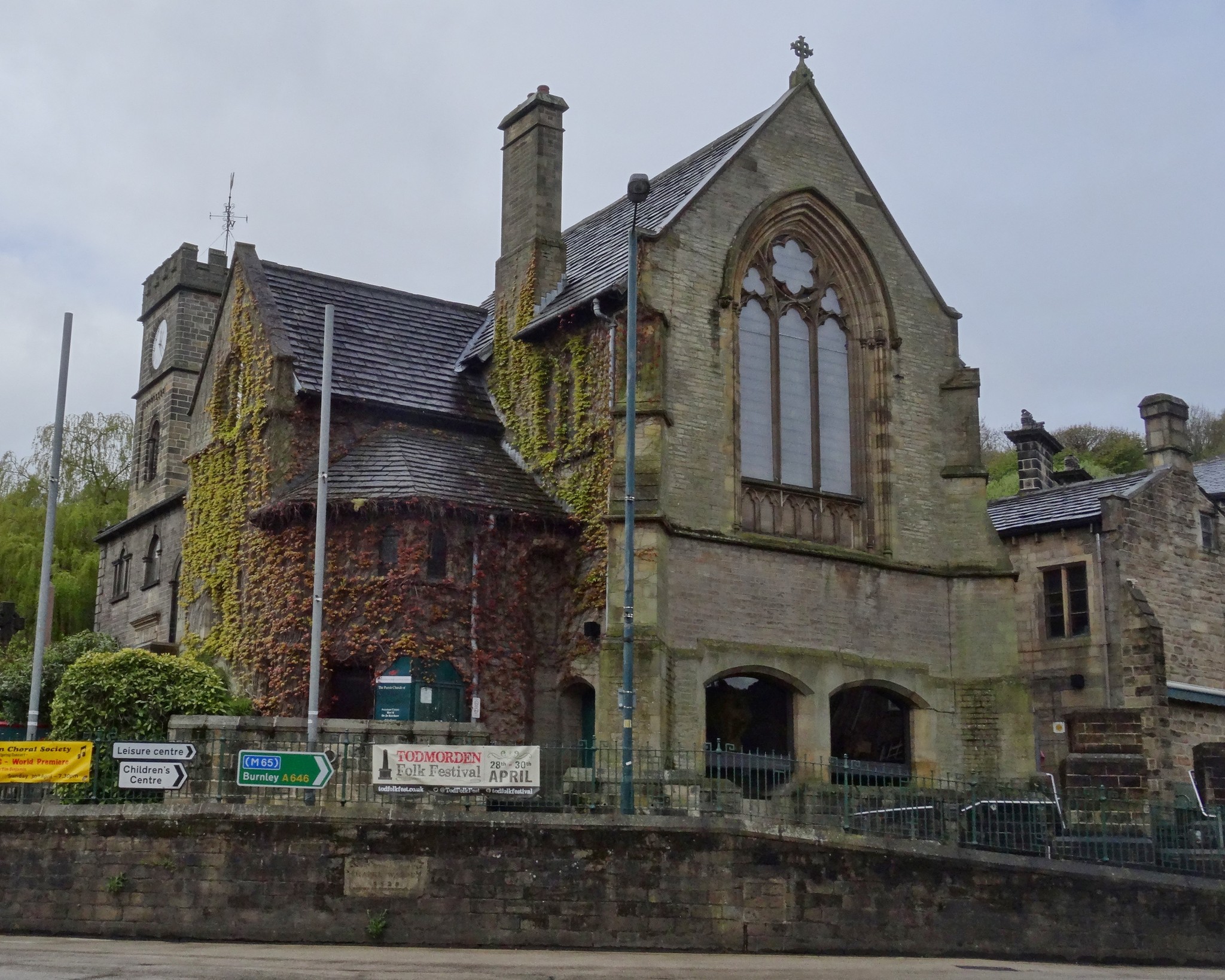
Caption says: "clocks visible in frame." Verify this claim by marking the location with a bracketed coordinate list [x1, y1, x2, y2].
[152, 319, 167, 370]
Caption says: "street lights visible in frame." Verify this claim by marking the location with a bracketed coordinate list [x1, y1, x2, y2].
[623, 172, 651, 813]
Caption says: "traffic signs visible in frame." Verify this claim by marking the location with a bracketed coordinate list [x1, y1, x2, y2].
[119, 762, 189, 791]
[238, 750, 333, 788]
[112, 742, 196, 761]
